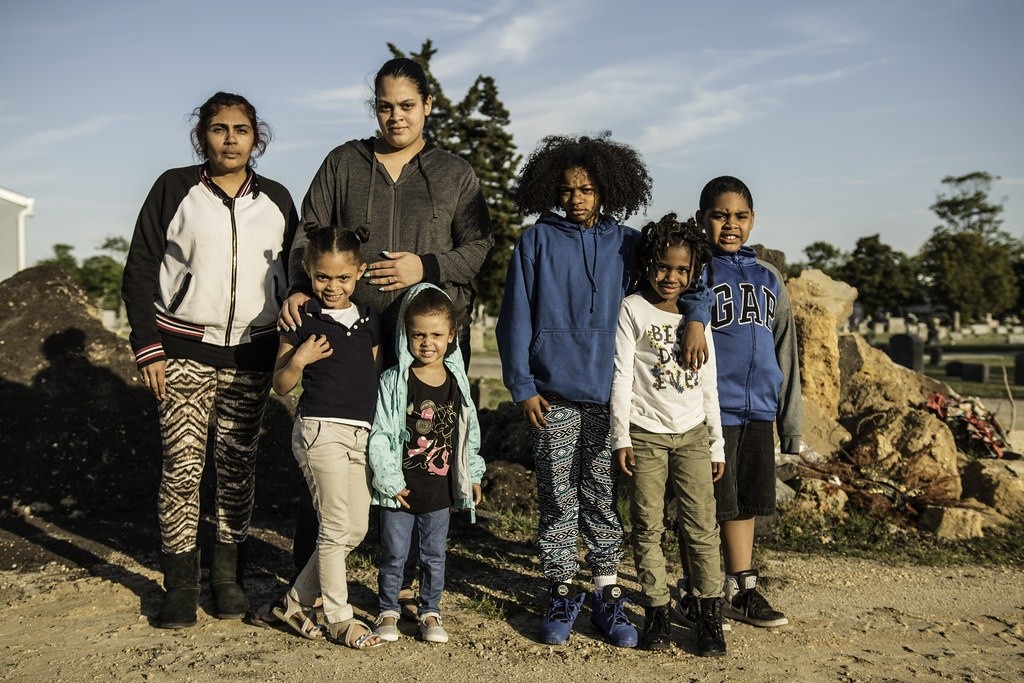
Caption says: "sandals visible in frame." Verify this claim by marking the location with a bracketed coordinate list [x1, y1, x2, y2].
[273, 594, 327, 640]
[326, 618, 385, 650]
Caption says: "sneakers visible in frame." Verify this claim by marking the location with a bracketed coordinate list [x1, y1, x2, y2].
[723, 569, 788, 627]
[592, 584, 639, 647]
[694, 596, 726, 656]
[640, 602, 670, 649]
[675, 579, 731, 630]
[539, 581, 586, 645]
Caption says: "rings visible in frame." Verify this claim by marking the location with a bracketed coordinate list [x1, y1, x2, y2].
[142, 379, 150, 382]
[388, 276, 397, 283]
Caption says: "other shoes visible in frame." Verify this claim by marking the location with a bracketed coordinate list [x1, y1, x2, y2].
[374, 610, 400, 641]
[417, 612, 448, 643]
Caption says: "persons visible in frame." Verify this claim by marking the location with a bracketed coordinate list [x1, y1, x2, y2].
[493, 133, 717, 646]
[277, 58, 495, 626]
[608, 212, 727, 657]
[676, 177, 801, 625]
[367, 282, 487, 644]
[271, 222, 388, 651]
[122, 93, 300, 628]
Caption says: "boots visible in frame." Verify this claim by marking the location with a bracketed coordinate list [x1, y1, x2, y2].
[208, 531, 252, 620]
[154, 542, 201, 629]
